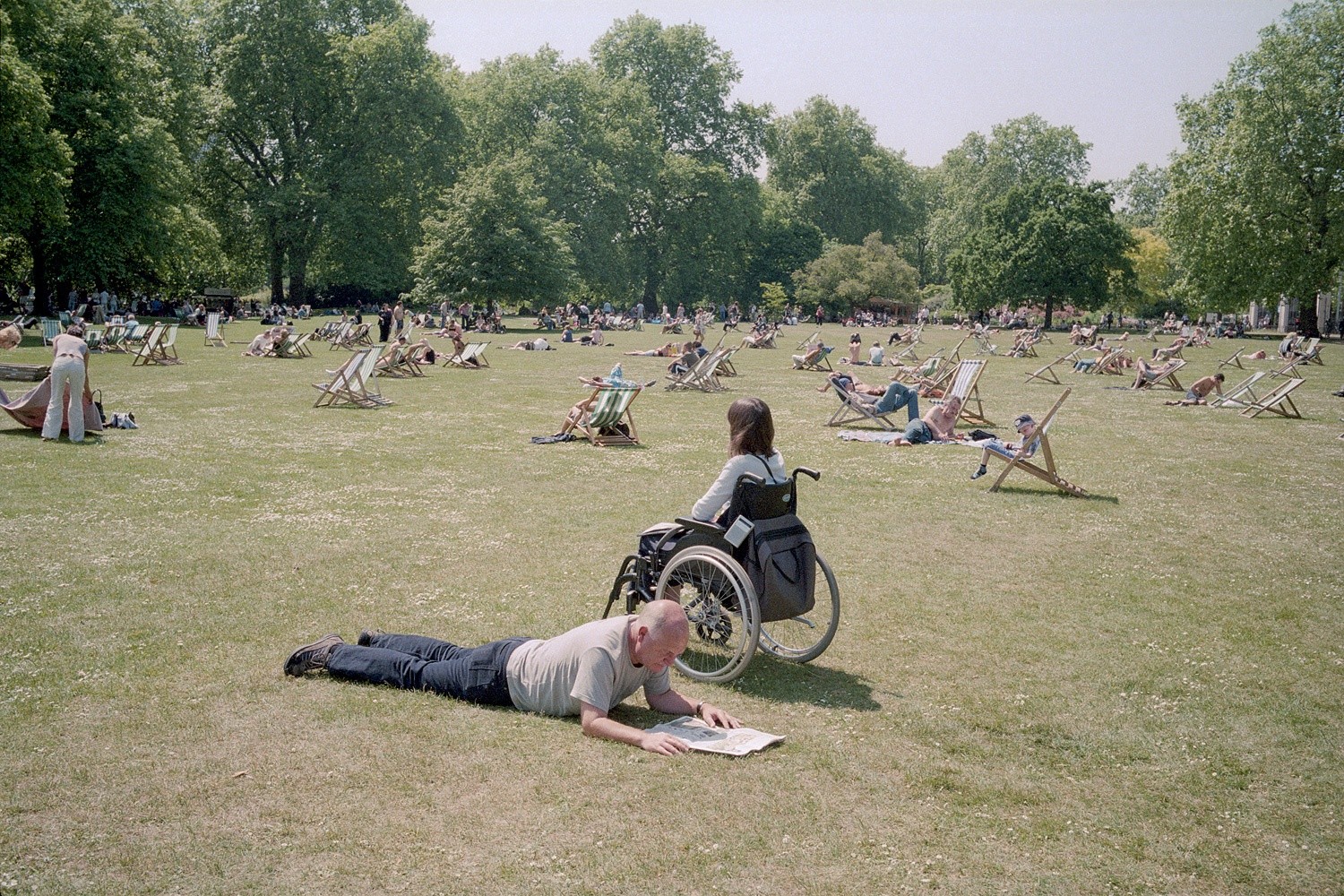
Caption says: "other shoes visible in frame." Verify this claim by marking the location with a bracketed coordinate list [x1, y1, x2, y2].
[648, 380, 656, 386]
[42, 437, 58, 443]
[550, 432, 564, 437]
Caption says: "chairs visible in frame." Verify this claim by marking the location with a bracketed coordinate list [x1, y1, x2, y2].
[0, 312, 1344, 496]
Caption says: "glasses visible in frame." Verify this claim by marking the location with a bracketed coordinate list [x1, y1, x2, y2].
[12, 340, 20, 348]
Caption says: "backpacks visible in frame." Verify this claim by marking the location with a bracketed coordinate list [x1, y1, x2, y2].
[736, 477, 817, 624]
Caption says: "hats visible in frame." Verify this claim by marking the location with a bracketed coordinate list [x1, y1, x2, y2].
[1015, 415, 1036, 433]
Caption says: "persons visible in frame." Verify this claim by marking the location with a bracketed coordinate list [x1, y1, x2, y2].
[0, 315, 40, 350]
[283, 599, 744, 757]
[41, 326, 91, 443]
[637, 397, 787, 604]
[66, 287, 1344, 480]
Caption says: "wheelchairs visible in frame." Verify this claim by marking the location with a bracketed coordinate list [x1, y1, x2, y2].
[602, 464, 841, 685]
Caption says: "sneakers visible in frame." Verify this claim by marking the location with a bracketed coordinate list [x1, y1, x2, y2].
[357, 628, 387, 647]
[283, 633, 348, 678]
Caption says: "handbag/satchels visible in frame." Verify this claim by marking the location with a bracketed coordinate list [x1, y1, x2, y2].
[598, 419, 631, 437]
[93, 388, 106, 424]
[973, 429, 997, 441]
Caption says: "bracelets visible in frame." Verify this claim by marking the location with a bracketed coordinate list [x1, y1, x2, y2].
[696, 700, 706, 715]
